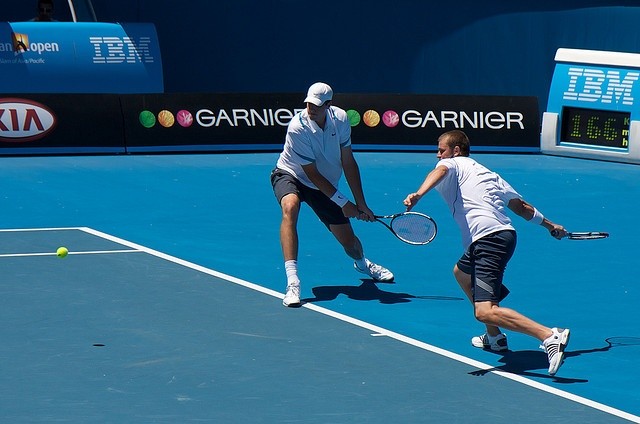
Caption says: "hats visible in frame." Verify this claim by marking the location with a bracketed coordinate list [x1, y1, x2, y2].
[304, 82, 333, 106]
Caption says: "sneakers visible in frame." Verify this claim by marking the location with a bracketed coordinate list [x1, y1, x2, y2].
[282, 282, 300, 306]
[539, 327, 570, 375]
[354, 257, 394, 281]
[472, 333, 508, 351]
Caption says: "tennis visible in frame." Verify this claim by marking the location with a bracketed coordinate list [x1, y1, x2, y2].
[57, 247, 69, 260]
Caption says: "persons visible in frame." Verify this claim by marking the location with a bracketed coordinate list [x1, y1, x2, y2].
[403, 130, 570, 375]
[270, 82, 394, 307]
